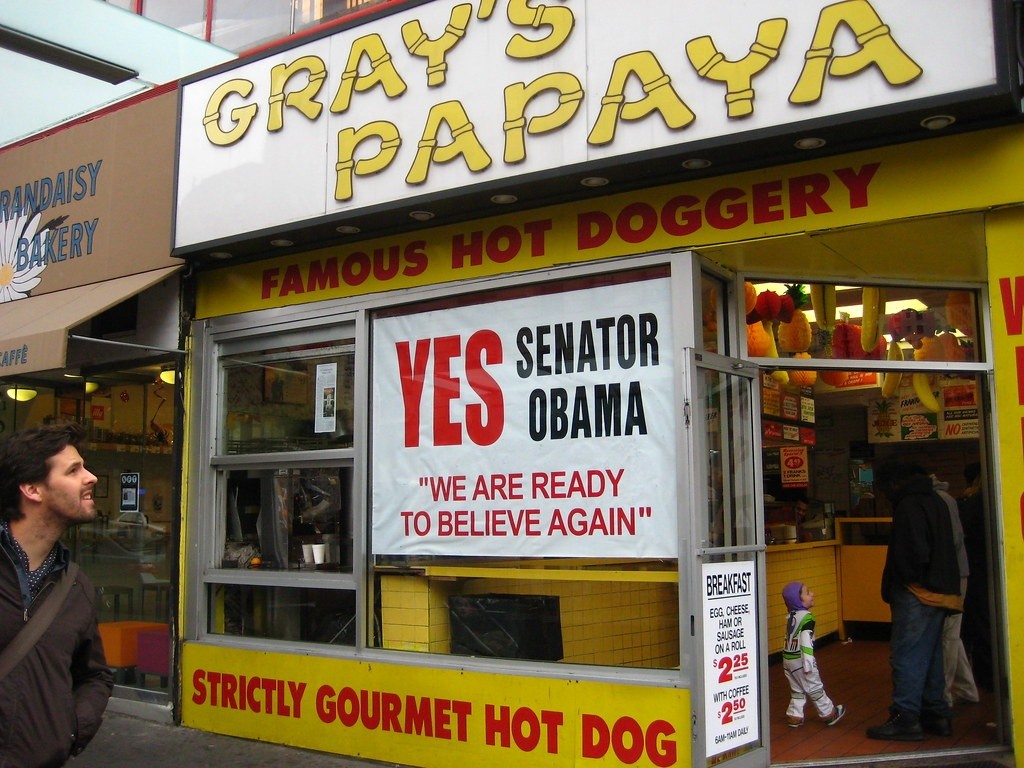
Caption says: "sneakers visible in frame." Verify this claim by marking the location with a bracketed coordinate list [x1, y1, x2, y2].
[866, 702, 924, 740]
[918, 713, 951, 736]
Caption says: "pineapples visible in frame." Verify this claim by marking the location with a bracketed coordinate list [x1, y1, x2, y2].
[790, 352, 817, 385]
[777, 283, 811, 353]
[913, 305, 944, 362]
[935, 318, 960, 361]
[745, 320, 771, 357]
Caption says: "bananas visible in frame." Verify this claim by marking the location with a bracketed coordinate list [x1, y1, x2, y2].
[811, 284, 836, 331]
[912, 372, 940, 412]
[882, 338, 905, 398]
[763, 323, 789, 384]
[861, 287, 886, 351]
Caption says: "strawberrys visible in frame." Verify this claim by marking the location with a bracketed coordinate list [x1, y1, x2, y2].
[779, 295, 794, 323]
[746, 308, 761, 325]
[756, 290, 781, 321]
[888, 309, 935, 341]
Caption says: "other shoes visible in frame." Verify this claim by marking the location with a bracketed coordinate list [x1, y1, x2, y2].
[825, 705, 845, 726]
[788, 719, 804, 727]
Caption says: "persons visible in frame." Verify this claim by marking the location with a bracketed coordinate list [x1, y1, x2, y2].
[778, 450, 993, 741]
[0, 425, 116, 768]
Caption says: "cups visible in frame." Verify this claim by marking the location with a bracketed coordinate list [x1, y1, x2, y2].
[302, 544, 313, 562]
[312, 545, 326, 564]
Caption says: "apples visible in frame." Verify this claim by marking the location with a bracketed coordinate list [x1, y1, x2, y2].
[832, 324, 865, 361]
[865, 336, 887, 360]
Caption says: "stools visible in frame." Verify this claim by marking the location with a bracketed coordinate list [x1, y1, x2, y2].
[95, 572, 171, 620]
[97, 620, 170, 690]
